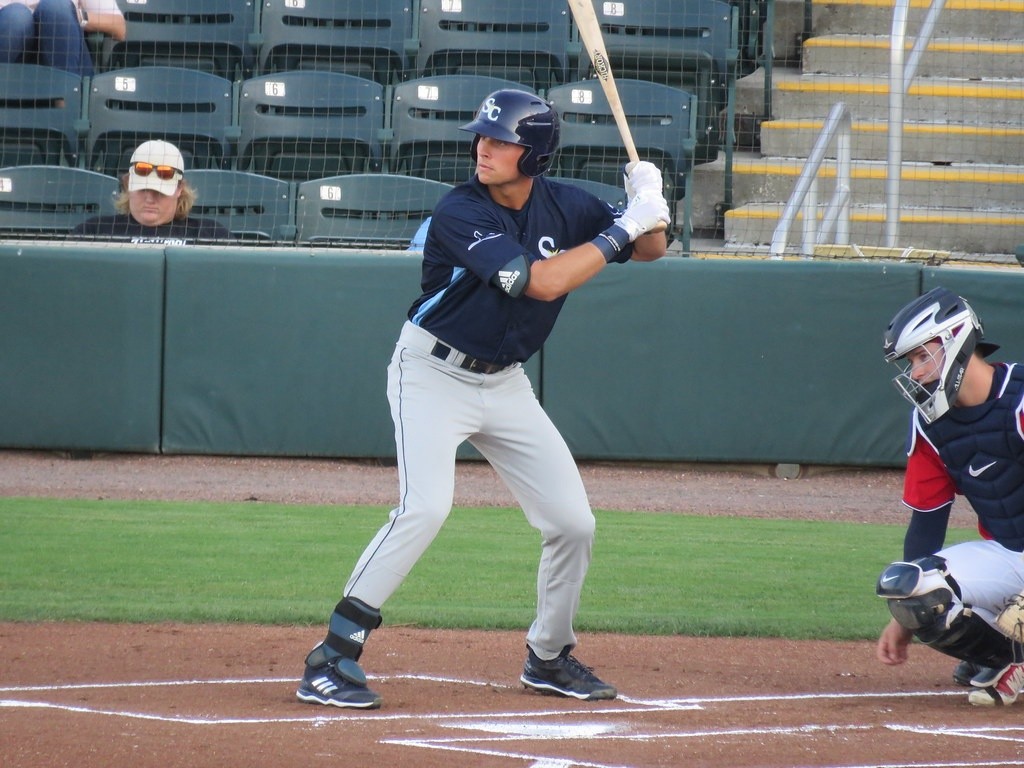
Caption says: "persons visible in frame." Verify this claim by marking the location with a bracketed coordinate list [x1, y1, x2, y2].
[0, 0, 126, 108]
[297, 89, 671, 708]
[874, 287, 1024, 706]
[65, 139, 239, 245]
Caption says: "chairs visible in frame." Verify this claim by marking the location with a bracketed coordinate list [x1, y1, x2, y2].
[174, 170, 293, 245]
[547, 77, 700, 257]
[84, 66, 237, 174]
[579, 0, 735, 164]
[386, 73, 537, 193]
[233, 69, 383, 186]
[556, 176, 629, 262]
[415, 2, 571, 85]
[253, 1, 417, 89]
[0, 166, 121, 239]
[109, 0, 253, 84]
[0, 61, 87, 170]
[295, 174, 459, 253]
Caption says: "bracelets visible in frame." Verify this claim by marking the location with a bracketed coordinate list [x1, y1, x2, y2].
[590, 224, 629, 263]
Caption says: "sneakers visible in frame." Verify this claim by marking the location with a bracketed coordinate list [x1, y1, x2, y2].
[519, 643, 618, 701]
[295, 665, 384, 710]
[968, 664, 1024, 707]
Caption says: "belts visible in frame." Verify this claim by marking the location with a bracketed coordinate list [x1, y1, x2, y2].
[431, 341, 517, 374]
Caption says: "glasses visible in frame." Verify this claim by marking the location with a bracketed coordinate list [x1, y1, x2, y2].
[129, 162, 184, 180]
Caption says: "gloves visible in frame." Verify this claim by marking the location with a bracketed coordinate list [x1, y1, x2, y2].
[623, 161, 662, 207]
[614, 190, 671, 243]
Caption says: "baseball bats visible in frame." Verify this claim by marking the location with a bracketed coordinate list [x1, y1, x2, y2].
[566, 1, 668, 235]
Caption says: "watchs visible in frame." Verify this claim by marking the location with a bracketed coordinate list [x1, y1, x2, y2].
[79, 9, 88, 28]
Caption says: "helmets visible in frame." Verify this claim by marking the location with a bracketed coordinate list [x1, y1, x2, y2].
[457, 88, 560, 178]
[882, 286, 986, 428]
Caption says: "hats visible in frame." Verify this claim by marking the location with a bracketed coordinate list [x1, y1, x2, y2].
[128, 139, 184, 196]
